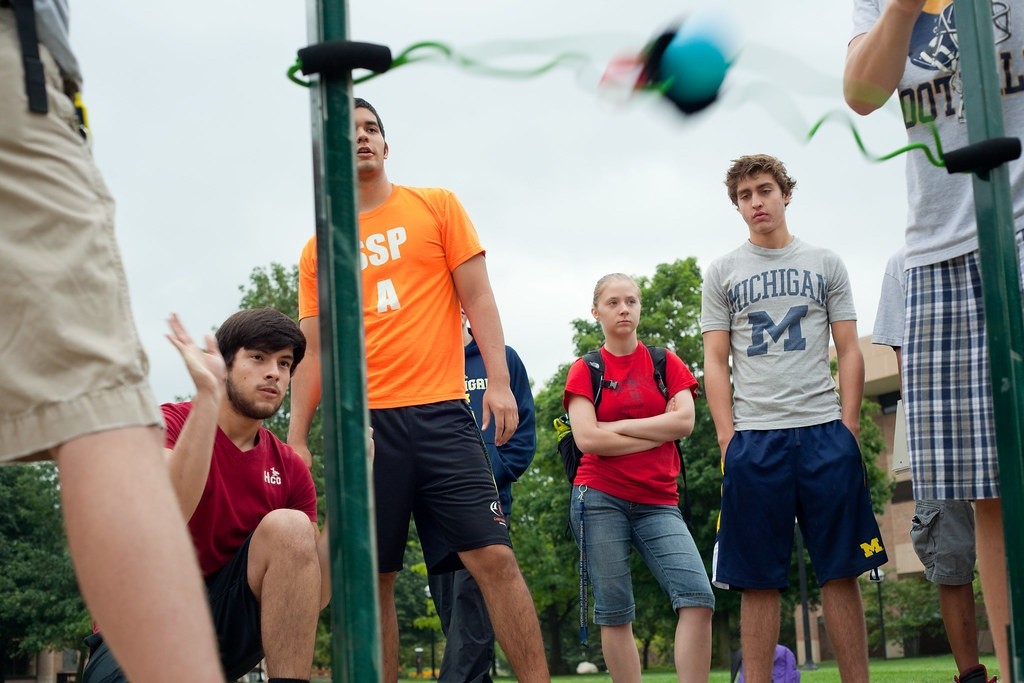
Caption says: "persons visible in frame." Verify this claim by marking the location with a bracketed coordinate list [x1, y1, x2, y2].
[865, 253, 992, 683]
[562, 274, 717, 683]
[407, 307, 535, 680]
[76, 310, 379, 680]
[845, 1, 1024, 683]
[0, 0, 225, 682]
[287, 95, 553, 682]
[699, 154, 887, 683]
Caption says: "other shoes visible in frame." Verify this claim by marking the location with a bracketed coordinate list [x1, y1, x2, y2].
[953, 662, 998, 683]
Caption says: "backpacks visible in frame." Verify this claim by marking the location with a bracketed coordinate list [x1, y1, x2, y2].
[559, 344, 668, 484]
